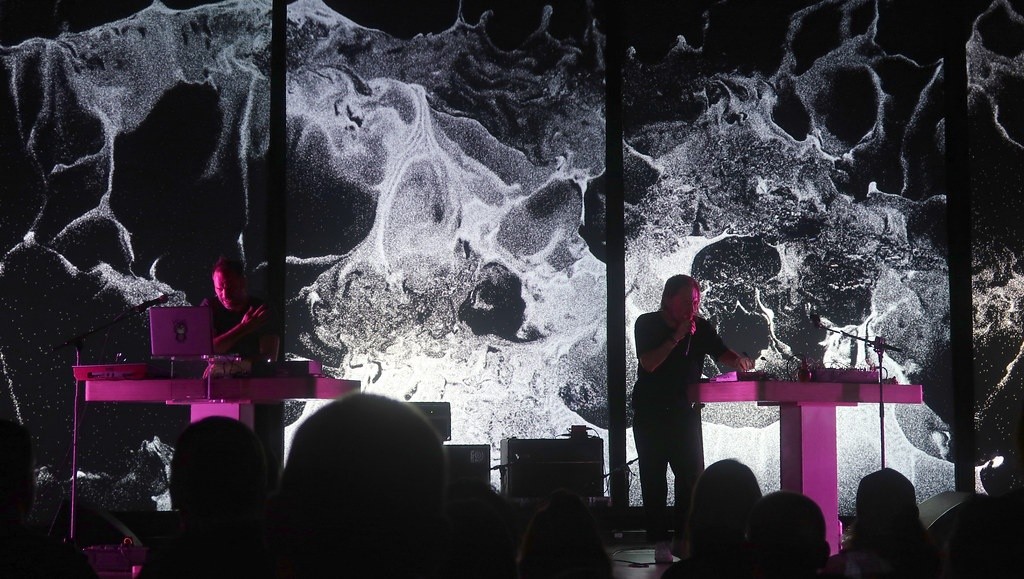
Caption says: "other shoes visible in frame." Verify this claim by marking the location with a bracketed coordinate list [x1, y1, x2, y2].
[654, 544, 674, 566]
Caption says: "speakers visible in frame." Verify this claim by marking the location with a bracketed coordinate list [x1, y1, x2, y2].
[48, 495, 144, 547]
[500, 439, 604, 497]
[403, 402, 452, 441]
[919, 490, 974, 530]
[444, 444, 490, 485]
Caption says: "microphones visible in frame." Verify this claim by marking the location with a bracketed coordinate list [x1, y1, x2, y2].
[682, 333, 693, 357]
[137, 294, 168, 309]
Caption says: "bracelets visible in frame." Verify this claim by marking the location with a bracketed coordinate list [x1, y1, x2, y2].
[671, 337, 677, 346]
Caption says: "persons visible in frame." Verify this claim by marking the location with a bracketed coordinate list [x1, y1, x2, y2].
[136, 395, 613, 578]
[0, 420, 98, 579]
[658, 459, 1024, 579]
[196, 255, 283, 464]
[632, 275, 755, 562]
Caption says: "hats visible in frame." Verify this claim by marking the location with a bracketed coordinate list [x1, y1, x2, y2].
[277, 393, 447, 530]
[169, 415, 269, 511]
[856, 468, 920, 520]
[690, 459, 763, 528]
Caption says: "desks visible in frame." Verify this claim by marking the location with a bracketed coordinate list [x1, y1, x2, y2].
[86, 378, 361, 430]
[699, 381, 924, 553]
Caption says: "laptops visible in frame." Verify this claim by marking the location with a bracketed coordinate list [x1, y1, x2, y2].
[149, 306, 229, 356]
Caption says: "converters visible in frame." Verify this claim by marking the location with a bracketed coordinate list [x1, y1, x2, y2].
[570, 425, 588, 439]
